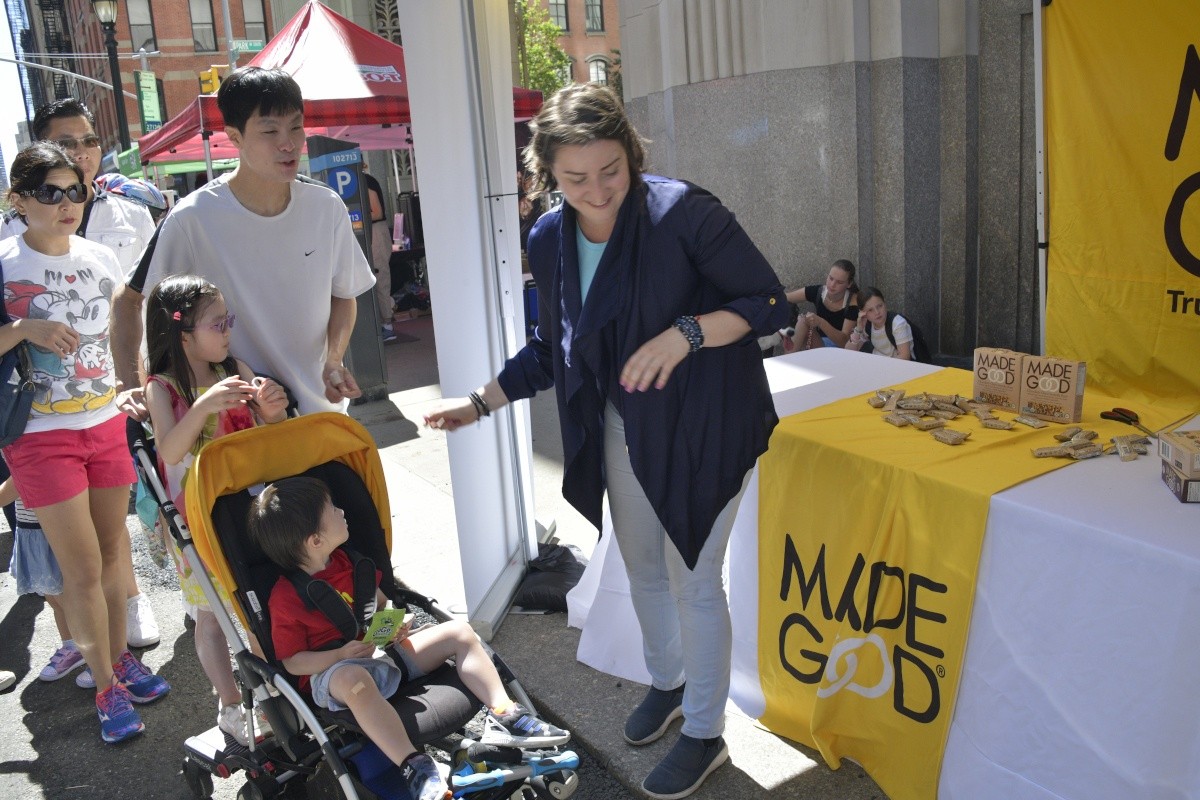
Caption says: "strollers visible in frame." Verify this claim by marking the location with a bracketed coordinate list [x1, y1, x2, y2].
[124, 371, 584, 800]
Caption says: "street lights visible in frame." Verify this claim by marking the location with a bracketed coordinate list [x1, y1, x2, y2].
[91, 0, 131, 152]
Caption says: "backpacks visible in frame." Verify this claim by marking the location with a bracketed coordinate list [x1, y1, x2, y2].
[865, 311, 931, 363]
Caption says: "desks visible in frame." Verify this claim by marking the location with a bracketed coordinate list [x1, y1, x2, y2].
[568, 342, 1200, 800]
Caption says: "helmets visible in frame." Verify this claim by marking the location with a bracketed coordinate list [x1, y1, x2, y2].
[111, 179, 168, 210]
[95, 173, 129, 193]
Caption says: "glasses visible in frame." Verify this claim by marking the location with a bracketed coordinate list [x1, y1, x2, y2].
[183, 313, 235, 333]
[57, 136, 101, 151]
[19, 182, 87, 205]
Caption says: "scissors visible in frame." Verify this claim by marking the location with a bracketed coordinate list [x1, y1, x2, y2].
[1100, 408, 1158, 438]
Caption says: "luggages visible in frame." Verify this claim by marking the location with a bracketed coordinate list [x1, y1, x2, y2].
[395, 189, 424, 243]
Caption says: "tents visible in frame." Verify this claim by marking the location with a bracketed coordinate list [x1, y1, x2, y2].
[118, 145, 312, 180]
[136, 0, 544, 184]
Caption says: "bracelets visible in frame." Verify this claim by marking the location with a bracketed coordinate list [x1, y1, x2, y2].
[670, 314, 704, 354]
[467, 390, 490, 422]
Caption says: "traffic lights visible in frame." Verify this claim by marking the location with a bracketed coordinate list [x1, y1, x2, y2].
[199, 67, 220, 94]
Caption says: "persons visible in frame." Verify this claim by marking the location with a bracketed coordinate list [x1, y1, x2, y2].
[844, 286, 917, 361]
[247, 476, 571, 800]
[143, 273, 289, 746]
[143, 64, 377, 423]
[782, 258, 864, 352]
[0, 97, 200, 743]
[357, 151, 398, 343]
[421, 78, 800, 798]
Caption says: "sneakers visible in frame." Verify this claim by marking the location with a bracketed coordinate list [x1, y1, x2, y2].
[216, 689, 275, 747]
[75, 667, 97, 688]
[112, 647, 172, 702]
[125, 591, 160, 647]
[623, 681, 685, 745]
[641, 733, 729, 800]
[39, 645, 87, 681]
[96, 672, 145, 742]
[479, 702, 571, 748]
[399, 752, 451, 800]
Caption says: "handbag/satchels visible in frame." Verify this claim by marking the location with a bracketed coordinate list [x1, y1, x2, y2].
[0, 261, 36, 446]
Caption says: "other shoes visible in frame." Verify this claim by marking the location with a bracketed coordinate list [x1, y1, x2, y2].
[381, 326, 397, 341]
[0, 667, 16, 691]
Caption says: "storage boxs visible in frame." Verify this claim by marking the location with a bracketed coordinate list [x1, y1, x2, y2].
[959, 344, 1091, 426]
[1145, 418, 1200, 510]
